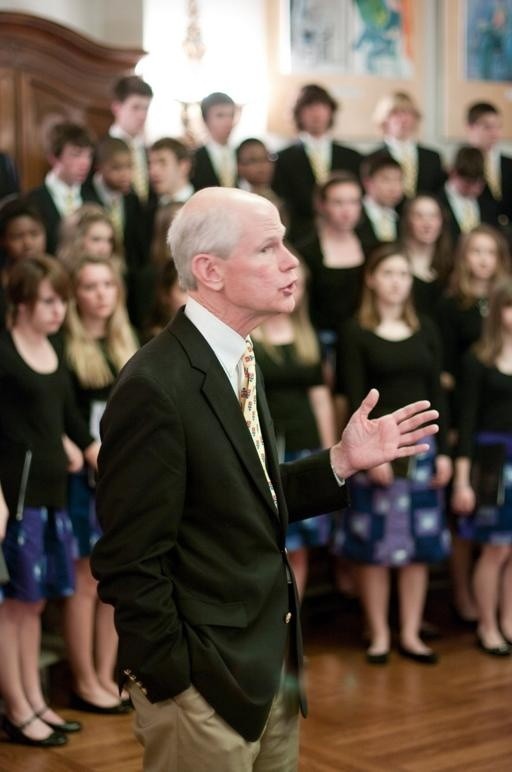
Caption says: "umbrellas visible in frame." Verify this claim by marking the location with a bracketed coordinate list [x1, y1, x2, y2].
[4, 715, 68, 748]
[450, 600, 483, 626]
[74, 683, 139, 715]
[367, 653, 389, 665]
[398, 638, 439, 664]
[38, 705, 82, 733]
[477, 633, 511, 656]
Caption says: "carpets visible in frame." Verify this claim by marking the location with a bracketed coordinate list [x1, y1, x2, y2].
[484, 154, 503, 203]
[107, 193, 126, 255]
[459, 198, 478, 236]
[311, 146, 329, 186]
[235, 339, 280, 510]
[64, 185, 83, 227]
[396, 145, 418, 198]
[214, 145, 236, 188]
[378, 208, 396, 245]
[125, 143, 148, 197]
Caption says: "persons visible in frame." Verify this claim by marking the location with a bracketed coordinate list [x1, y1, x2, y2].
[6, 122, 511, 592]
[0, 251, 86, 749]
[270, 84, 371, 243]
[186, 92, 250, 194]
[325, 243, 453, 667]
[465, 102, 512, 215]
[246, 243, 337, 612]
[439, 222, 510, 629]
[83, 186, 440, 772]
[365, 92, 449, 202]
[94, 76, 158, 225]
[450, 279, 511, 654]
[57, 242, 145, 717]
